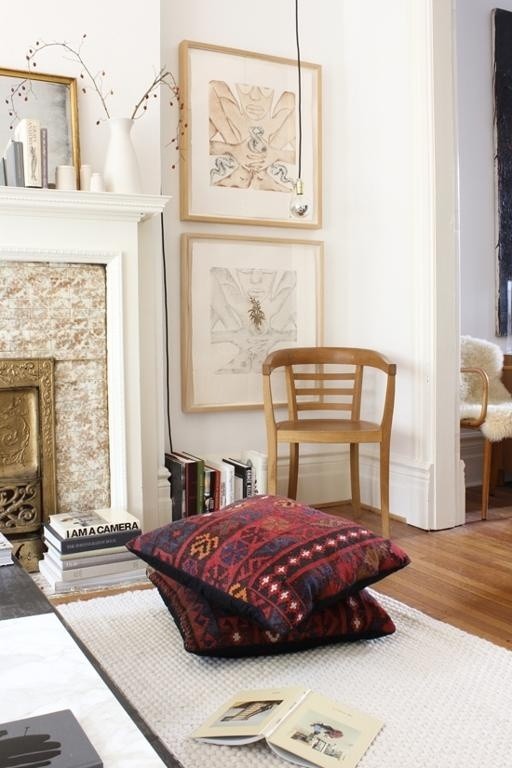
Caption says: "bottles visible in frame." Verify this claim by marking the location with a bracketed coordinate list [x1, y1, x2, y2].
[80, 163, 105, 194]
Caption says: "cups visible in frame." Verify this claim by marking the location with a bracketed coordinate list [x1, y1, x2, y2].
[54, 165, 77, 190]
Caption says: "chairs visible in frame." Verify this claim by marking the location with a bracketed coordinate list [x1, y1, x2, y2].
[262, 346, 396, 543]
[459, 335, 512, 519]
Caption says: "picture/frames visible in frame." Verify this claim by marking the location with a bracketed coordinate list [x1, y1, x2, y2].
[177, 40, 323, 230]
[180, 233, 323, 415]
[0, 67, 81, 192]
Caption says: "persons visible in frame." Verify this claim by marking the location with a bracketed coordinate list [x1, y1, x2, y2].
[208, 80, 299, 193]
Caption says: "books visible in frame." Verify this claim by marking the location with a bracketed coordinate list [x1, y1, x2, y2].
[40, 126, 49, 190]
[189, 685, 386, 768]
[0, 531, 13, 566]
[3, 139, 26, 188]
[37, 506, 150, 592]
[164, 448, 269, 522]
[12, 118, 44, 188]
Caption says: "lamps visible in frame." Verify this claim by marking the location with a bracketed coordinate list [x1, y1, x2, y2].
[291, 0, 312, 218]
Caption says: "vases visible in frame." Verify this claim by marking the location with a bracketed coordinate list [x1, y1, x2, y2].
[104, 119, 139, 194]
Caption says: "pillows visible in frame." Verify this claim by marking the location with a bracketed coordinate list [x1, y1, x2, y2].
[141, 566, 394, 656]
[123, 493, 408, 639]
